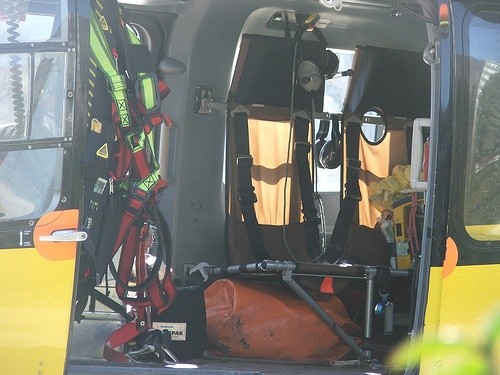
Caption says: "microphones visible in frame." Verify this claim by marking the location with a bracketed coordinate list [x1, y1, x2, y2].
[331, 69, 353, 79]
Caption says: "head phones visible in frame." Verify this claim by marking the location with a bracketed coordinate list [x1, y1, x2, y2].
[316, 114, 344, 168]
[294, 25, 338, 94]
